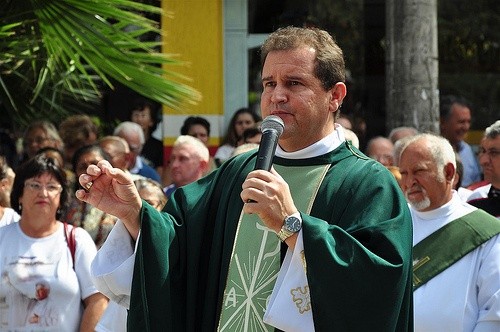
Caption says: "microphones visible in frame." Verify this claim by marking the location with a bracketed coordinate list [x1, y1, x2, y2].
[247, 114, 285, 204]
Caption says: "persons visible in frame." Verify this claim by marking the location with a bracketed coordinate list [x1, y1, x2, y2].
[74, 23, 416, 332]
[0, 97, 111, 332]
[365, 92, 499, 332]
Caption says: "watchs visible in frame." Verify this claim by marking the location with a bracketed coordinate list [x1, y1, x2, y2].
[277, 211, 303, 242]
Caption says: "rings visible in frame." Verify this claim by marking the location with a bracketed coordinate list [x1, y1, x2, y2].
[84, 182, 92, 191]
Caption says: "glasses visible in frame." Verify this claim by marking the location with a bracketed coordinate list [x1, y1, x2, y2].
[25, 181, 64, 196]
